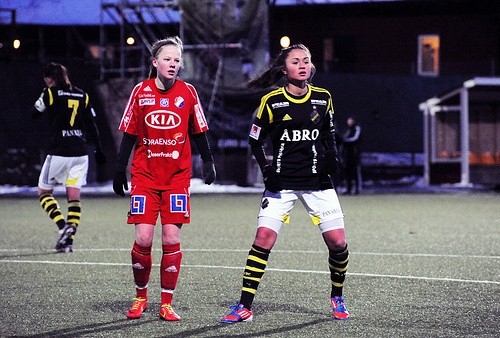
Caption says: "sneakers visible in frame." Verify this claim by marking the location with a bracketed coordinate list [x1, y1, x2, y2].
[220, 304, 253, 323]
[55, 226, 76, 250]
[331, 296, 350, 320]
[159, 304, 181, 321]
[57, 246, 72, 252]
[127, 297, 147, 318]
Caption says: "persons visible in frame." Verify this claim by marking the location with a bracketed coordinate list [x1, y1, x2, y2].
[30, 63, 107, 253]
[332, 116, 362, 196]
[115, 37, 217, 320]
[220, 44, 349, 324]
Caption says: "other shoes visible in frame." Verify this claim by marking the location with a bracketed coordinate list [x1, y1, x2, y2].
[355, 190, 361, 194]
[342, 191, 352, 195]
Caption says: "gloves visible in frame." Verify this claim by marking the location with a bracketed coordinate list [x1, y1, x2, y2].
[263, 166, 283, 193]
[113, 172, 128, 197]
[203, 161, 216, 186]
[333, 156, 342, 175]
